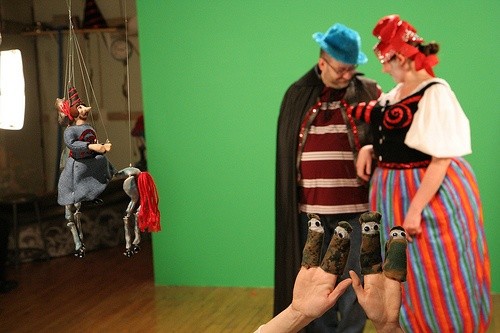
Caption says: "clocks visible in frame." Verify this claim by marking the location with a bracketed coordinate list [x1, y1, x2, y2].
[110, 38, 134, 66]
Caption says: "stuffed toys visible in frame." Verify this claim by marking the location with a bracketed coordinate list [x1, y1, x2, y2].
[320, 221, 353, 275]
[359, 211, 383, 275]
[384, 226, 407, 281]
[302, 213, 325, 268]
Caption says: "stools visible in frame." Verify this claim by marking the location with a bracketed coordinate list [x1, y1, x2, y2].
[0, 193, 51, 286]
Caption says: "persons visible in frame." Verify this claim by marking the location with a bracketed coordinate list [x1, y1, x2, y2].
[253, 211, 408, 333]
[354, 14, 493, 333]
[272, 22, 384, 333]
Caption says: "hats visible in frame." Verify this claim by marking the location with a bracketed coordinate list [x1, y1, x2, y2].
[371, 14, 438, 80]
[312, 25, 368, 66]
